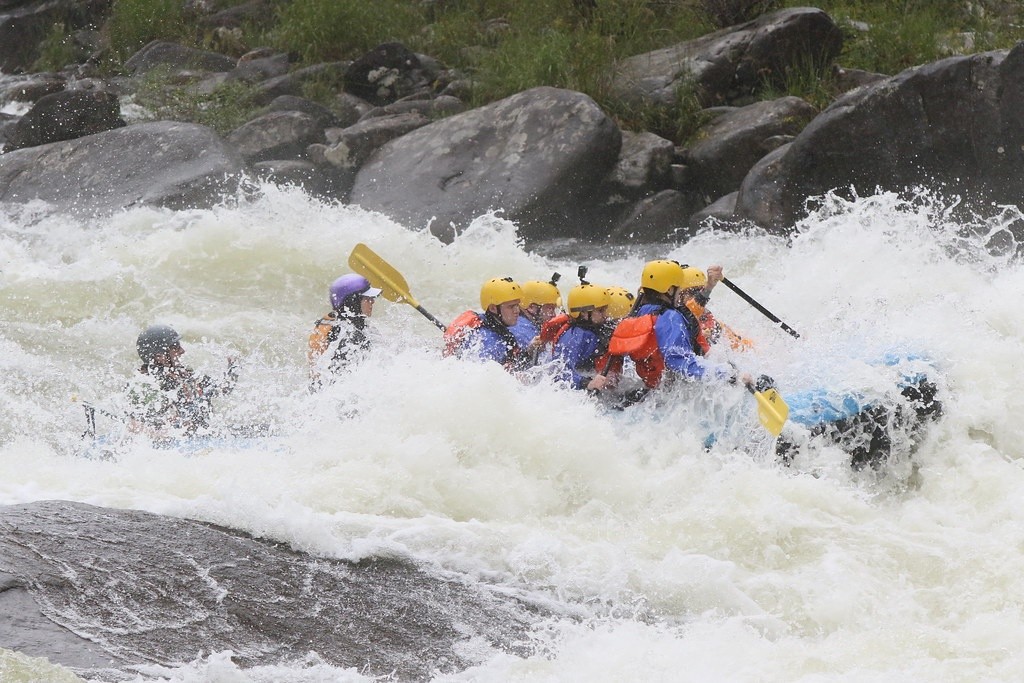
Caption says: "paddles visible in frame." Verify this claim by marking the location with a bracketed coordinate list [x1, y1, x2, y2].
[720, 274, 800, 339]
[347, 242, 445, 330]
[729, 359, 788, 436]
[589, 291, 643, 397]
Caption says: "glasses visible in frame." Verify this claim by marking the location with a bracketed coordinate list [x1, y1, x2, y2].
[169, 342, 180, 353]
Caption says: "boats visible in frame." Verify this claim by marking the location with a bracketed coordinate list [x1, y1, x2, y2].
[709, 348, 950, 485]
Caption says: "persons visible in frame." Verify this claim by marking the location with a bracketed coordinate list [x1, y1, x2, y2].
[591, 287, 634, 389]
[611, 260, 756, 411]
[445, 276, 543, 373]
[123, 324, 240, 440]
[552, 286, 610, 395]
[508, 281, 561, 351]
[679, 265, 753, 355]
[310, 274, 382, 377]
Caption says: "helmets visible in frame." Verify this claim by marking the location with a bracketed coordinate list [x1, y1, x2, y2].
[567, 284, 610, 318]
[136, 325, 183, 364]
[641, 260, 684, 293]
[480, 277, 523, 311]
[680, 267, 706, 288]
[329, 274, 370, 309]
[519, 280, 563, 310]
[606, 285, 636, 319]
